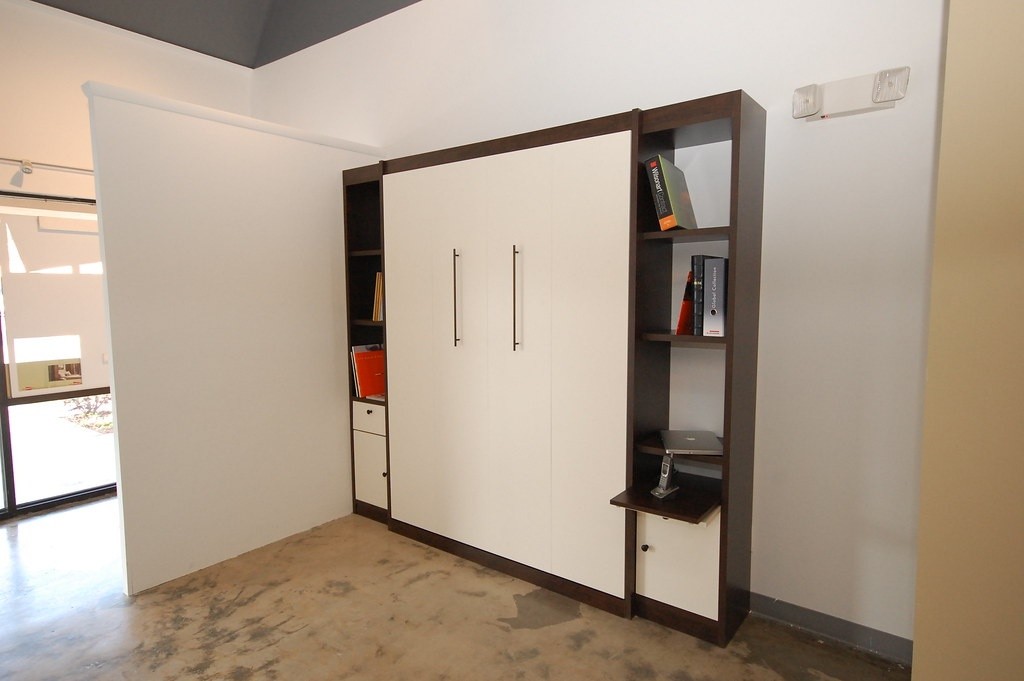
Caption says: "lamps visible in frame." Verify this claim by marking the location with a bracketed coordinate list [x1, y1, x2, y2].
[22, 159, 33, 174]
[791, 65, 910, 121]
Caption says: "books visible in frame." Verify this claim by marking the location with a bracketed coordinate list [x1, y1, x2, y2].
[645, 154, 698, 232]
[373, 272, 384, 322]
[675, 255, 725, 337]
[350, 344, 386, 402]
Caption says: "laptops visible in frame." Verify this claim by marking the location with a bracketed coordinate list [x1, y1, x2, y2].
[660, 429, 723, 455]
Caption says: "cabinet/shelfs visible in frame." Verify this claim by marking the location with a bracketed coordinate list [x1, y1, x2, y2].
[378, 105, 670, 620]
[610, 83, 763, 646]
[343, 163, 389, 528]
